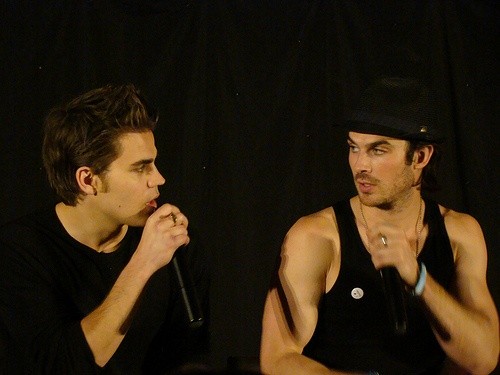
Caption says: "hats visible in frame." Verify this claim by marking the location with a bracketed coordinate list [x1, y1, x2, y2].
[331, 76, 452, 141]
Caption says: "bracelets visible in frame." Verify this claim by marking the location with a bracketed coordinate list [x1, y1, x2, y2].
[401, 262, 427, 307]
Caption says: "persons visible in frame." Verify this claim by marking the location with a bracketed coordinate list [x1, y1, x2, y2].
[0, 84, 208, 375]
[259, 75, 500, 375]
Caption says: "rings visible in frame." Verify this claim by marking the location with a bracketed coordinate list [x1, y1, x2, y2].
[381, 237, 386, 246]
[169, 213, 177, 222]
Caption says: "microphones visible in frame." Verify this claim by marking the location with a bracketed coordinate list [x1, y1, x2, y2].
[385, 263, 407, 331]
[171, 244, 204, 329]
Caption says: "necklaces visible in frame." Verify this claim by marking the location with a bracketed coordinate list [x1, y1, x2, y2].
[359, 199, 422, 287]
